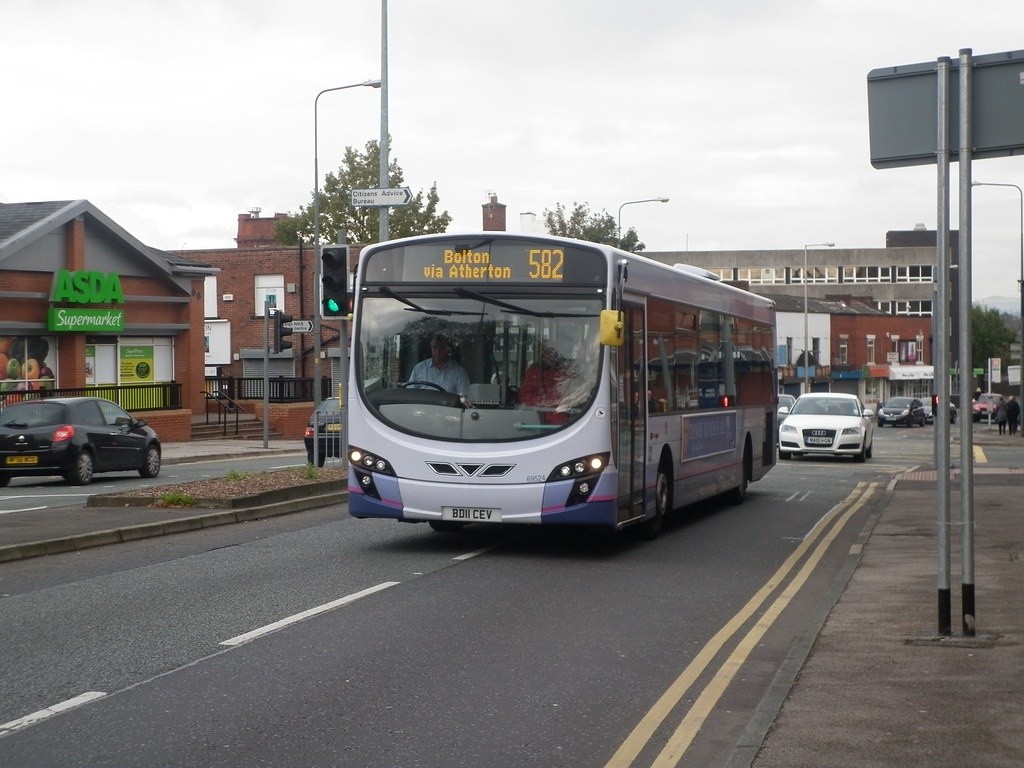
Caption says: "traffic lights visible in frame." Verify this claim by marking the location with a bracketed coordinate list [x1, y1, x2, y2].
[318, 242, 355, 322]
[272, 309, 294, 355]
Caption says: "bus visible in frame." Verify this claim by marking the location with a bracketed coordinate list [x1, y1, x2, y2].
[341, 227, 780, 541]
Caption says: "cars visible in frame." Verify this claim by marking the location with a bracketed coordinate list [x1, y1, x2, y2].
[777, 393, 797, 442]
[972, 393, 1006, 421]
[922, 397, 958, 424]
[777, 392, 875, 462]
[0, 396, 163, 486]
[876, 396, 925, 427]
[303, 396, 339, 469]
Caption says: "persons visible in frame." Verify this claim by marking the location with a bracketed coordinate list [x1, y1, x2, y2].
[404, 334, 471, 394]
[519, 338, 578, 424]
[995, 395, 1021, 436]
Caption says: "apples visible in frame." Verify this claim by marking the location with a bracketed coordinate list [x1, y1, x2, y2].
[0, 335, 54, 406]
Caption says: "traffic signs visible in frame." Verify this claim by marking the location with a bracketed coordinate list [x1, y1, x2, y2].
[283, 319, 312, 332]
[350, 187, 413, 207]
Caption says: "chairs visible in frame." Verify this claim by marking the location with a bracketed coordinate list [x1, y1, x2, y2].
[452, 334, 492, 385]
[839, 403, 852, 415]
[827, 406, 839, 414]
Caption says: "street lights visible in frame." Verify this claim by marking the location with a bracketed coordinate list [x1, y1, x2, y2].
[803, 241, 835, 393]
[309, 78, 381, 409]
[617, 197, 671, 249]
[971, 181, 1024, 438]
